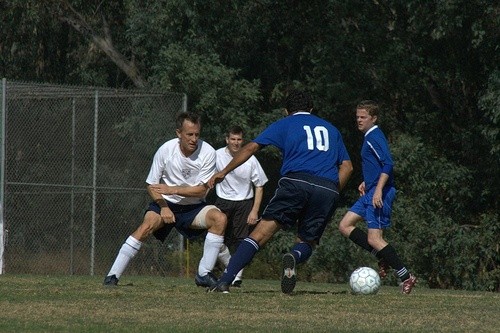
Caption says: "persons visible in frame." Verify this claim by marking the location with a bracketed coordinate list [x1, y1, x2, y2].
[216, 125, 268, 285]
[103, 111, 227, 287]
[208, 91, 353, 294]
[340, 100, 416, 295]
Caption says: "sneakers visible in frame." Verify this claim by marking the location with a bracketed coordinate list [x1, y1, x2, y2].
[402, 273, 416, 294]
[195, 271, 219, 287]
[206, 280, 230, 293]
[377, 258, 391, 279]
[281, 251, 297, 293]
[103, 274, 119, 286]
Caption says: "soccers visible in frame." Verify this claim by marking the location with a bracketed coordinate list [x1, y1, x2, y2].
[349, 266, 380, 296]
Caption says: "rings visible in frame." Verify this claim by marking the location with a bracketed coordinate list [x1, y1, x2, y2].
[377, 201, 379, 202]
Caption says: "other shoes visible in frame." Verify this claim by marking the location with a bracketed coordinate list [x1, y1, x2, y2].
[232, 276, 242, 285]
